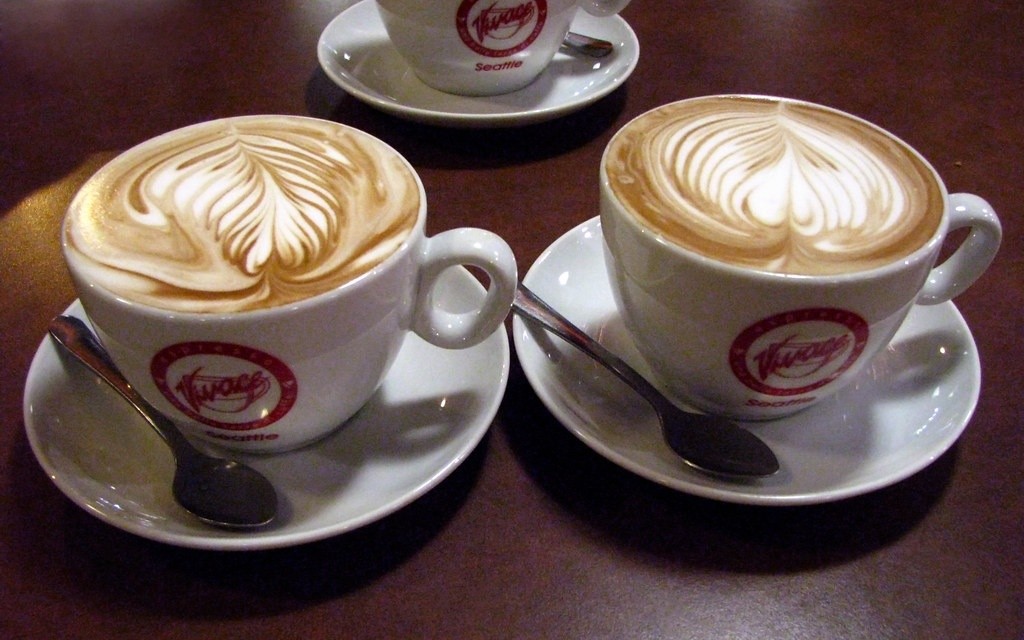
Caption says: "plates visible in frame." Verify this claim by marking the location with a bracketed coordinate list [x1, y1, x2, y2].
[316, 1, 640, 128]
[23, 265, 510, 550]
[512, 215, 981, 505]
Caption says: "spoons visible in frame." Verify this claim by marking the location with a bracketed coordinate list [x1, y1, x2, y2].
[513, 282, 780, 478]
[48, 315, 278, 527]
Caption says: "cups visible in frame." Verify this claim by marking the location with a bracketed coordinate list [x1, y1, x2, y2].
[61, 115, 518, 455]
[376, 0, 632, 96]
[600, 94, 1002, 422]
